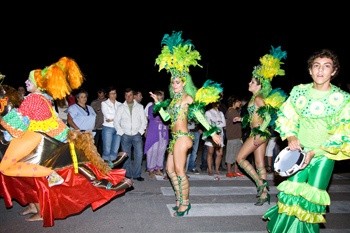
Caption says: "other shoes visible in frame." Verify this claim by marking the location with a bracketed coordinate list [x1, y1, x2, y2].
[157, 171, 163, 176]
[235, 172, 245, 176]
[133, 177, 144, 181]
[47, 172, 64, 187]
[226, 173, 236, 177]
[145, 169, 149, 173]
[208, 171, 214, 176]
[215, 170, 221, 174]
[222, 165, 226, 170]
[186, 173, 190, 178]
[267, 166, 272, 172]
[202, 168, 206, 172]
[163, 176, 169, 180]
[149, 172, 154, 177]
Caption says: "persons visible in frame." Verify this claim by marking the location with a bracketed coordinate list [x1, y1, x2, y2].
[145, 90, 164, 118]
[145, 90, 169, 177]
[263, 50, 350, 233]
[265, 136, 279, 172]
[149, 31, 224, 217]
[0, 57, 134, 228]
[184, 96, 245, 179]
[237, 45, 288, 206]
[113, 91, 147, 181]
[132, 88, 144, 108]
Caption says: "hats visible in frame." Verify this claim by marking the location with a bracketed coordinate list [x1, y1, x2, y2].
[252, 45, 287, 87]
[155, 31, 201, 77]
[30, 57, 83, 100]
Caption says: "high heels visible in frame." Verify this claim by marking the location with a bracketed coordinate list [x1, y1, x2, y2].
[255, 192, 270, 206]
[173, 202, 191, 216]
[257, 179, 269, 196]
[173, 206, 180, 210]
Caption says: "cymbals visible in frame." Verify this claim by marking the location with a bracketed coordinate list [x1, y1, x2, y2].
[275, 169, 280, 176]
[290, 170, 293, 174]
[276, 157, 280, 163]
[301, 145, 304, 150]
[287, 148, 290, 150]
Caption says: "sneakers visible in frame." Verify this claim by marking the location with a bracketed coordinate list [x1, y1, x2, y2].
[190, 168, 200, 174]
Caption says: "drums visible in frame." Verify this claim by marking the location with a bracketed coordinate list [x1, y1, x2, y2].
[273, 145, 305, 177]
